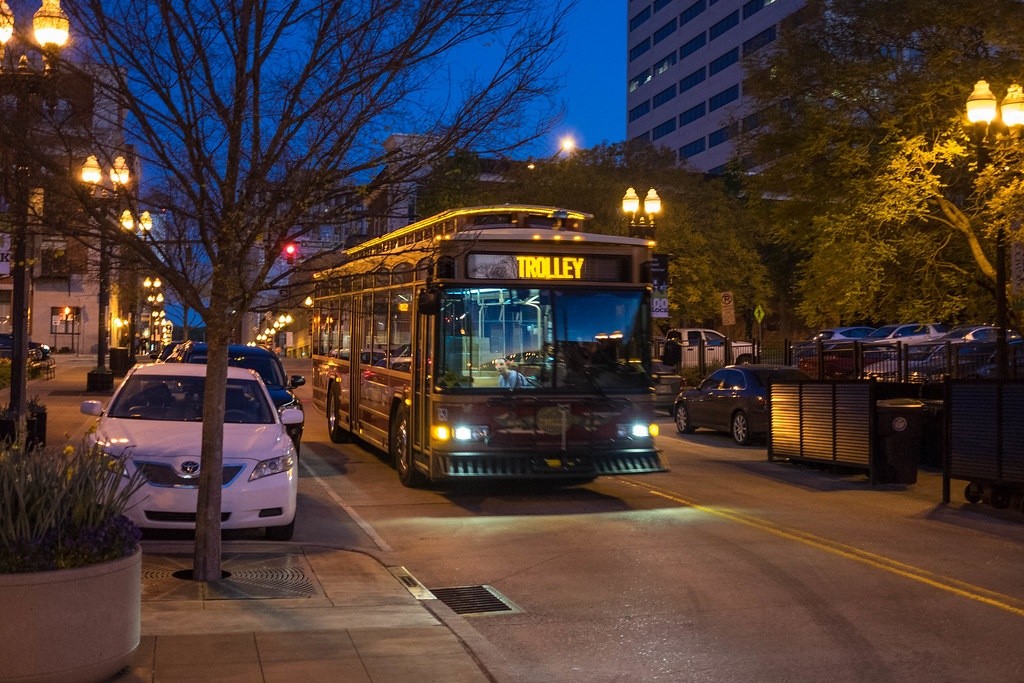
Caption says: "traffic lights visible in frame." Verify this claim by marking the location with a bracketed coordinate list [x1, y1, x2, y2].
[286, 245, 295, 264]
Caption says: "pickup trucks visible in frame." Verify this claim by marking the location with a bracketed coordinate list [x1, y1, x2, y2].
[652, 329, 763, 368]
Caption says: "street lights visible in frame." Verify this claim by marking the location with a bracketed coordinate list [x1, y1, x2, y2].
[121, 210, 153, 365]
[622, 187, 661, 242]
[143, 277, 167, 357]
[81, 154, 129, 394]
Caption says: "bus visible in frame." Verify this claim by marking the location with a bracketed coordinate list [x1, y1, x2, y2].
[310, 203, 672, 489]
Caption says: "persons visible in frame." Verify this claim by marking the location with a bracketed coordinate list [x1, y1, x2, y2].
[143, 381, 171, 408]
[566, 342, 612, 385]
[375, 344, 386, 359]
[494, 358, 528, 387]
[665, 332, 682, 373]
[135, 335, 147, 355]
[362, 343, 370, 363]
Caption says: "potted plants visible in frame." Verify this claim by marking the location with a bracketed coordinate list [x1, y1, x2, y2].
[0, 413, 144, 683]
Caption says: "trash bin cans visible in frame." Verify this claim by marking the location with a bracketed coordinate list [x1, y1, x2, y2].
[870, 397, 927, 489]
[109, 346, 128, 371]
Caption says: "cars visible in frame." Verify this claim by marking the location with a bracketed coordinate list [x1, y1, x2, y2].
[617, 358, 685, 414]
[0, 332, 51, 361]
[792, 323, 1024, 384]
[330, 343, 411, 373]
[671, 363, 816, 444]
[80, 363, 304, 541]
[150, 341, 185, 361]
[163, 340, 307, 464]
